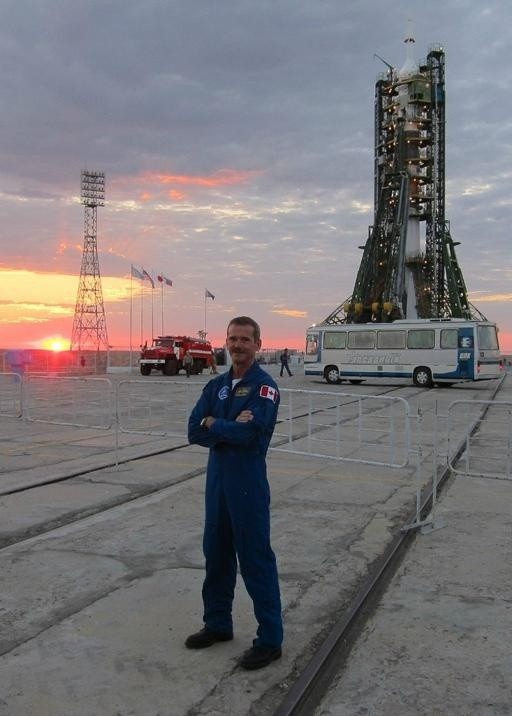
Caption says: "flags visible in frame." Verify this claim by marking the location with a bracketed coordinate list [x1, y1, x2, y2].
[131, 266, 146, 282]
[141, 269, 155, 290]
[151, 270, 167, 289]
[158, 270, 173, 287]
[205, 288, 216, 301]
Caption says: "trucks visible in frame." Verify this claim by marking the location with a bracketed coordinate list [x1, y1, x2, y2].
[137, 335, 217, 376]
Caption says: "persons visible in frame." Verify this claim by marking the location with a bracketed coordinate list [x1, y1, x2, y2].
[183, 314, 284, 670]
[81, 355, 86, 367]
[182, 350, 195, 379]
[279, 348, 294, 378]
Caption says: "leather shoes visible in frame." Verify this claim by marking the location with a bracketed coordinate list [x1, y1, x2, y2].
[241, 643, 282, 671]
[185, 625, 234, 650]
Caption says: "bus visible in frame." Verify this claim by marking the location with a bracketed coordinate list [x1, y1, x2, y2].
[303, 317, 504, 388]
